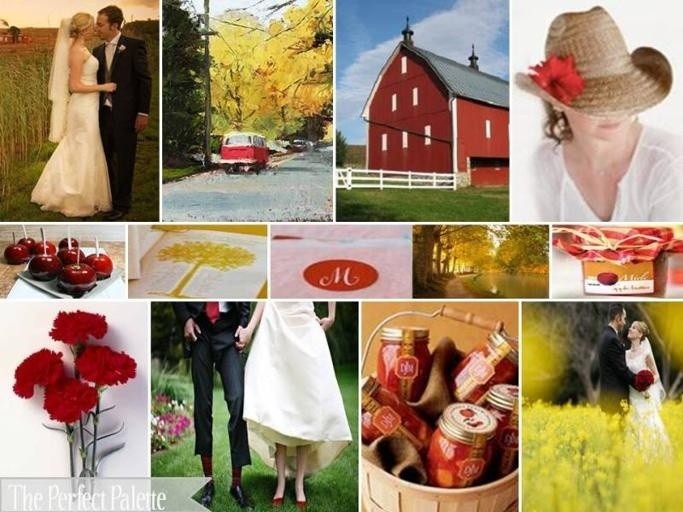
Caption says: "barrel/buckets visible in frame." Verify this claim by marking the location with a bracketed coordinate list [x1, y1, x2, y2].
[361, 305, 519, 512]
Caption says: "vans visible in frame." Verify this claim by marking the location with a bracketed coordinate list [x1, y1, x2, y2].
[218, 131, 269, 176]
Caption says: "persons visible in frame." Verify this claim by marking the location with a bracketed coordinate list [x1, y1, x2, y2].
[596, 305, 635, 416]
[92, 5, 152, 222]
[515, 7, 682, 221]
[624, 320, 669, 443]
[174, 302, 252, 509]
[29, 12, 117, 218]
[240, 302, 354, 506]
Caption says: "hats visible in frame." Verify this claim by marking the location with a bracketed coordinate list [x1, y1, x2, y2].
[517, 7, 673, 121]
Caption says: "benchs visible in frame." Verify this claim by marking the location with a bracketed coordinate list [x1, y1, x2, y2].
[1, 34, 23, 44]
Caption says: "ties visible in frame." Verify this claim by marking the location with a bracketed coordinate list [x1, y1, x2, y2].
[206, 302, 219, 324]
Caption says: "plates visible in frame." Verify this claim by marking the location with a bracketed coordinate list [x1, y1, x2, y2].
[15, 266, 121, 298]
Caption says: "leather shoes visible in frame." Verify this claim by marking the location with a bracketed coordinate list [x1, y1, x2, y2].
[202, 484, 213, 506]
[230, 485, 254, 511]
[297, 500, 306, 507]
[272, 498, 282, 505]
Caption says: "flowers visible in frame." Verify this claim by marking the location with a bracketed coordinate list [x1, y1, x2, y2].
[12, 310, 137, 500]
[529, 56, 583, 107]
[635, 371, 655, 398]
